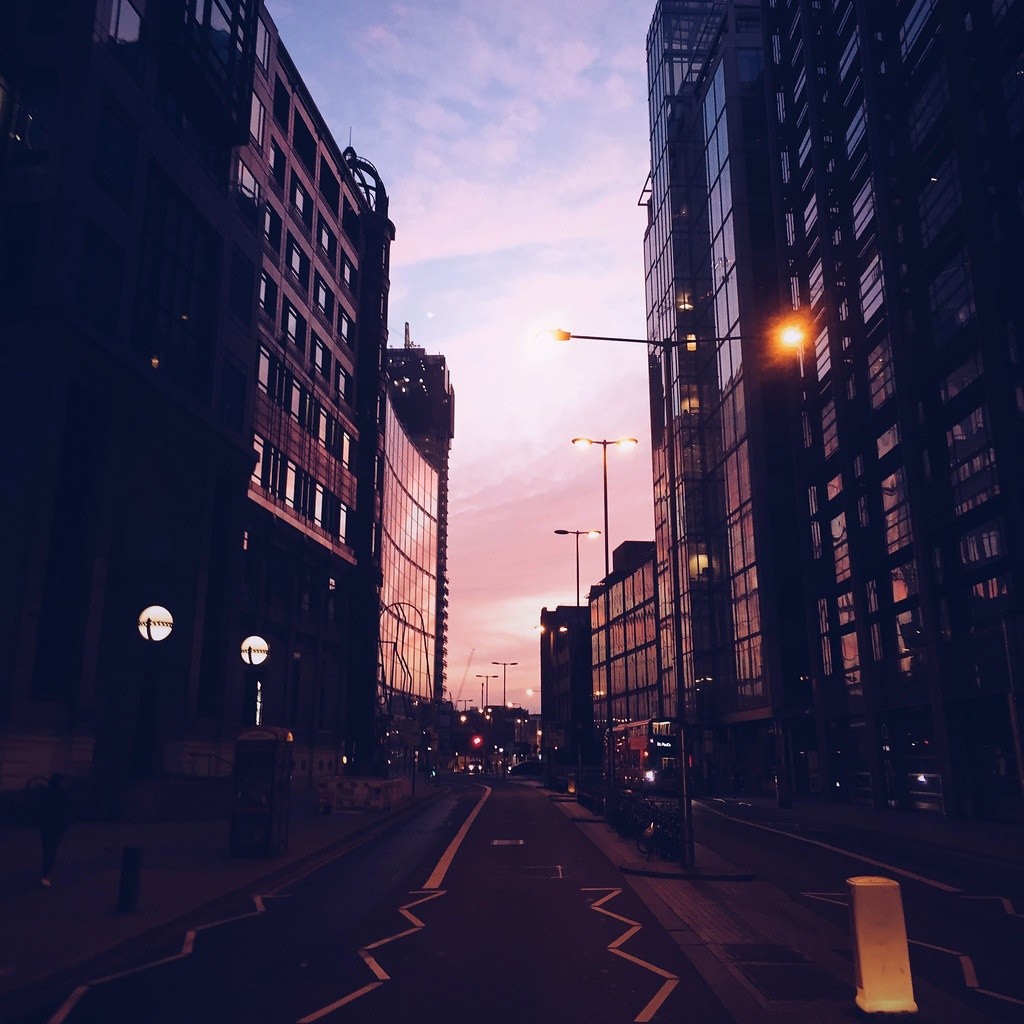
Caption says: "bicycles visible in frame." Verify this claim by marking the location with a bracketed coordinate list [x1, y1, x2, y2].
[615, 792, 685, 862]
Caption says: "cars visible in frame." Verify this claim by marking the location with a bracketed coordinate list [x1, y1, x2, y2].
[511, 761, 543, 776]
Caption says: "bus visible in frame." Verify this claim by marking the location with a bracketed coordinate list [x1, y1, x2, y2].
[602, 717, 683, 791]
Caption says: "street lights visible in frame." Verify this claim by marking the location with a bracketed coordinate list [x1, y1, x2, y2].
[549, 326, 806, 866]
[458, 700, 473, 711]
[554, 530, 602, 787]
[240, 635, 270, 732]
[116, 604, 173, 915]
[535, 626, 568, 730]
[572, 439, 637, 786]
[492, 662, 518, 709]
[476, 675, 498, 707]
